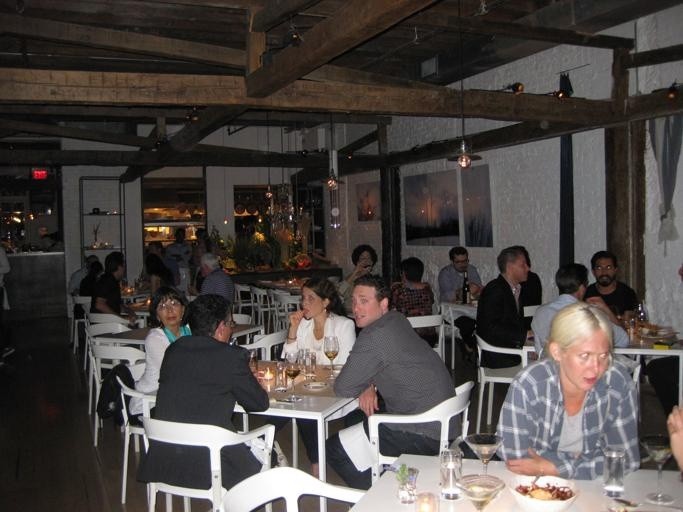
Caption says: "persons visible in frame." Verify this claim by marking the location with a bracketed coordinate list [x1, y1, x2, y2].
[334, 244, 378, 305]
[140, 240, 181, 286]
[166, 228, 192, 295]
[476, 247, 529, 368]
[0, 245, 15, 367]
[582, 251, 638, 329]
[190, 246, 206, 295]
[667, 405, 683, 473]
[74, 261, 103, 320]
[494, 302, 641, 480]
[90, 252, 138, 329]
[69, 255, 99, 294]
[259, 278, 357, 480]
[438, 246, 482, 341]
[128, 286, 192, 428]
[325, 275, 462, 490]
[136, 294, 277, 490]
[531, 263, 589, 359]
[389, 256, 434, 316]
[200, 254, 234, 303]
[142, 253, 176, 292]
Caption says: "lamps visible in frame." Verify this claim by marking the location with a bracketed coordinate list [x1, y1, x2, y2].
[318, 113, 340, 189]
[447, 62, 482, 166]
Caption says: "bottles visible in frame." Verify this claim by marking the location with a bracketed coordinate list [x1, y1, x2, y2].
[461, 270, 471, 304]
[635, 304, 645, 321]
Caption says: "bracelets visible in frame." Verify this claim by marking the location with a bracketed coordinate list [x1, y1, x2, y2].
[287, 336, 298, 340]
[538, 463, 546, 477]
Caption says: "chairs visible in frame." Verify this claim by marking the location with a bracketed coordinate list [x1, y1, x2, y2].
[232, 313, 251, 344]
[219, 467, 367, 512]
[91, 346, 151, 445]
[114, 366, 153, 504]
[142, 399, 276, 512]
[88, 324, 135, 414]
[232, 283, 302, 335]
[83, 315, 144, 371]
[230, 329, 288, 432]
[474, 332, 544, 435]
[406, 315, 447, 364]
[368, 382, 473, 485]
[64, 295, 92, 354]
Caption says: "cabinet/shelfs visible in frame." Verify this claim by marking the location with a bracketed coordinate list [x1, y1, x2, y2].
[78, 175, 127, 288]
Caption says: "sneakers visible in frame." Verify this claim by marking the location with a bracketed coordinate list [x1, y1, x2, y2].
[2, 348, 15, 357]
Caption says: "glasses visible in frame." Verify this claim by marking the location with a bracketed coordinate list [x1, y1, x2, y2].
[453, 259, 469, 264]
[224, 321, 236, 329]
[594, 265, 613, 271]
[157, 301, 181, 311]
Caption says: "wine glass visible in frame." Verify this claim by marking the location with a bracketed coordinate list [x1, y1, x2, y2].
[323, 336, 340, 379]
[284, 353, 302, 401]
[456, 475, 506, 512]
[464, 433, 506, 473]
[639, 434, 676, 505]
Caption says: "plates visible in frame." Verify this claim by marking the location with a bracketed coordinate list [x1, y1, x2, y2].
[638, 333, 674, 339]
[302, 382, 330, 392]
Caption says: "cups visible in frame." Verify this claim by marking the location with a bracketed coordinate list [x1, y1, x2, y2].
[298, 349, 310, 374]
[397, 468, 419, 504]
[602, 446, 625, 497]
[440, 450, 462, 500]
[275, 366, 287, 392]
[304, 352, 317, 381]
[247, 349, 258, 374]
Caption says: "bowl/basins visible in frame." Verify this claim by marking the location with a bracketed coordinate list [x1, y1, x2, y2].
[509, 476, 579, 510]
